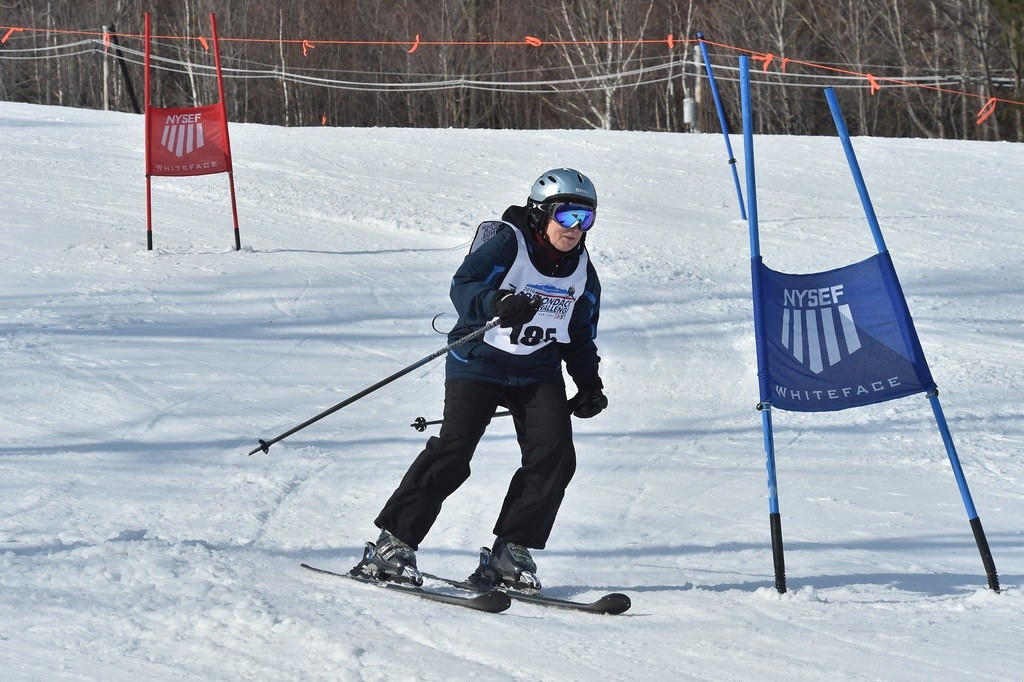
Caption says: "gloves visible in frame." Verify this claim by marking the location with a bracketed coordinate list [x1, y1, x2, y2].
[493, 289, 536, 329]
[572, 376, 604, 418]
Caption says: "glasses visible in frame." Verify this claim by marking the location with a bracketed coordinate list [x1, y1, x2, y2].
[547, 201, 597, 233]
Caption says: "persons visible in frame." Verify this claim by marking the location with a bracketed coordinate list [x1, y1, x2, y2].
[347, 167, 609, 591]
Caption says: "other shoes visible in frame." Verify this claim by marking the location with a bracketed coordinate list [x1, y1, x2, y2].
[375, 525, 417, 572]
[492, 533, 538, 579]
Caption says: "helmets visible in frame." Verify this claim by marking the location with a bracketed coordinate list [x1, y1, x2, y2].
[526, 167, 598, 213]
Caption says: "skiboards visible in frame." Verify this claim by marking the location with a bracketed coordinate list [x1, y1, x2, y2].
[300, 557, 635, 617]
